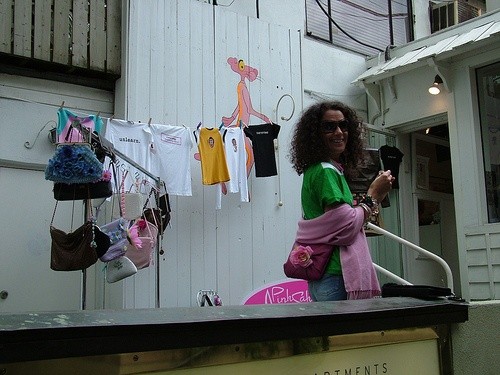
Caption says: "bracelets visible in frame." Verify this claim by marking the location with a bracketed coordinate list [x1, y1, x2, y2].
[359, 195, 378, 219]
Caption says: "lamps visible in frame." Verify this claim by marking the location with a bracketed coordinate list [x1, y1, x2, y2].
[428, 73, 443, 95]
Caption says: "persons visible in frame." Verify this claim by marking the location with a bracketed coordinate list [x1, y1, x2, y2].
[290, 101, 395, 302]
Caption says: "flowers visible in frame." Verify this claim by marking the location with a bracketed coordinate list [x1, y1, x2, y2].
[289, 245, 313, 269]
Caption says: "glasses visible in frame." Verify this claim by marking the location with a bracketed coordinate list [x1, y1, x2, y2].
[325, 119, 351, 131]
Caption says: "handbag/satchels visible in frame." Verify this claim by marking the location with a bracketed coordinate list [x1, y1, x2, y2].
[50, 200, 98, 272]
[44, 124, 111, 182]
[53, 127, 112, 201]
[128, 176, 169, 269]
[106, 256, 138, 284]
[283, 239, 336, 280]
[97, 171, 127, 261]
